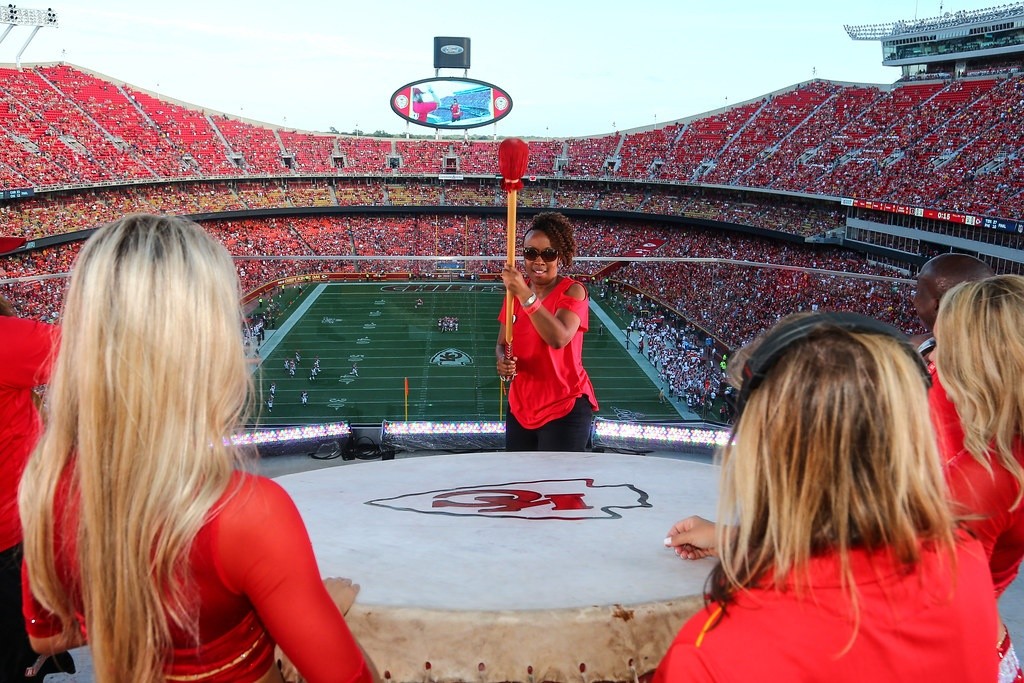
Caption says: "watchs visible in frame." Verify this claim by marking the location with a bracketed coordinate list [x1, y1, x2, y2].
[521, 292, 536, 306]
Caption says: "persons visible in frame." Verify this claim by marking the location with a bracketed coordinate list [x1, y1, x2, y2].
[496, 211, 599, 452]
[654, 311, 1000, 683]
[0, 292, 62, 683]
[0, 61, 1024, 417]
[928, 274, 1024, 599]
[16, 214, 382, 683]
[914, 253, 996, 465]
[450, 99, 461, 122]
[412, 85, 440, 122]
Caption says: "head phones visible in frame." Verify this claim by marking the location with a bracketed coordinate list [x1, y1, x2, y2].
[733, 309, 932, 405]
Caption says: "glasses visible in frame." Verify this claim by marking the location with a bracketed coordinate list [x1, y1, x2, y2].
[523, 246, 558, 262]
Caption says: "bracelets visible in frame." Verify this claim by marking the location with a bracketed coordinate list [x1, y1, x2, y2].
[525, 298, 541, 315]
[431, 91, 433, 93]
